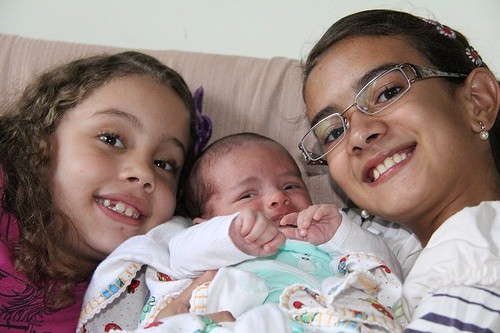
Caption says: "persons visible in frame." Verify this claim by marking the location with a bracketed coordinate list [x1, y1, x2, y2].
[0, 54, 235, 333]
[296, 10, 500, 333]
[170, 128, 403, 333]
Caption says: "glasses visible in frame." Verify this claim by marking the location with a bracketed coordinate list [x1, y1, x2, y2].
[299, 62, 468, 165]
